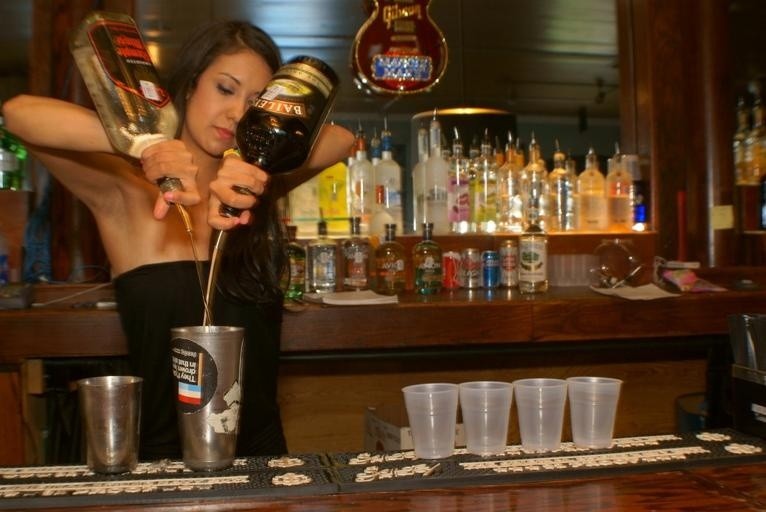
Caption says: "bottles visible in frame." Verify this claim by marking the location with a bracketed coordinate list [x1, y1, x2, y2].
[371, 224, 405, 299]
[0, 114, 27, 191]
[411, 220, 444, 294]
[731, 94, 766, 189]
[347, 119, 647, 231]
[66, 9, 187, 207]
[759, 175, 766, 230]
[279, 221, 305, 299]
[517, 197, 550, 297]
[217, 53, 341, 224]
[303, 216, 340, 296]
[340, 217, 371, 292]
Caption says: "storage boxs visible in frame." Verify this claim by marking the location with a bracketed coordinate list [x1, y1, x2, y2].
[363, 405, 466, 453]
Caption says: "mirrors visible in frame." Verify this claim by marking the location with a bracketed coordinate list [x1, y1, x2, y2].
[51, 1, 697, 230]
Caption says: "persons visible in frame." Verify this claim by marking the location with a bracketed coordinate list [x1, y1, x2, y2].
[2, 16, 355, 457]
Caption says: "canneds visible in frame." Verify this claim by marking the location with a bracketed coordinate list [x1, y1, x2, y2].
[460, 248, 480, 289]
[442, 250, 460, 291]
[480, 250, 501, 289]
[500, 240, 518, 288]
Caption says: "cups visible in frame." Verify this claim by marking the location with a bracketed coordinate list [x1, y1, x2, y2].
[459, 379, 516, 455]
[172, 323, 249, 475]
[74, 375, 147, 479]
[550, 253, 596, 288]
[511, 374, 571, 455]
[400, 381, 461, 462]
[561, 374, 624, 450]
[722, 312, 766, 370]
